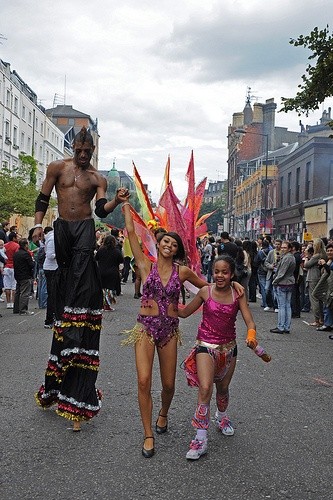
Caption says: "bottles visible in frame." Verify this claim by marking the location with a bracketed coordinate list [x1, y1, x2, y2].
[245, 339, 272, 363]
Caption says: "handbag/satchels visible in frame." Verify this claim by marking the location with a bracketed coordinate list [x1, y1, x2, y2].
[312, 270, 333, 306]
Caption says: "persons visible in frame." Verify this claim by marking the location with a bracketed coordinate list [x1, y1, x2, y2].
[10, 226, 18, 233]
[0, 239, 8, 274]
[117, 187, 245, 458]
[95, 229, 333, 312]
[13, 238, 35, 315]
[4, 232, 21, 309]
[270, 242, 296, 333]
[28, 227, 44, 299]
[0, 221, 10, 244]
[44, 227, 53, 234]
[316, 244, 333, 331]
[0, 254, 7, 302]
[177, 255, 257, 461]
[304, 237, 329, 326]
[43, 221, 58, 329]
[37, 236, 47, 308]
[23, 238, 34, 261]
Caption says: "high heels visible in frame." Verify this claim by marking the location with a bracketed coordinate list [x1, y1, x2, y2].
[142, 434, 154, 458]
[155, 409, 168, 434]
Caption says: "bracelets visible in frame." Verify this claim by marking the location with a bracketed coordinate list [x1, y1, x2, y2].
[232, 281, 234, 287]
[32, 125, 132, 325]
[32, 224, 42, 228]
[121, 203, 130, 209]
[115, 196, 121, 203]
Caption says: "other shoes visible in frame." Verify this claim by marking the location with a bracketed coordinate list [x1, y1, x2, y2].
[300, 307, 309, 311]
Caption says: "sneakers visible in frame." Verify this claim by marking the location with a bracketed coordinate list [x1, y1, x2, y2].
[100, 304, 115, 311]
[5, 302, 14, 309]
[311, 320, 323, 326]
[43, 320, 55, 329]
[270, 327, 289, 334]
[314, 324, 331, 332]
[12, 309, 33, 315]
[262, 306, 279, 313]
[186, 441, 209, 460]
[215, 414, 235, 436]
[0, 297, 5, 303]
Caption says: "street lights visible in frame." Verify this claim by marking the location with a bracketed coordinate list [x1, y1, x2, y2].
[233, 128, 270, 232]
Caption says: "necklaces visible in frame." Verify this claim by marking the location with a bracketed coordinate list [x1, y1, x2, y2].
[72, 157, 91, 183]
